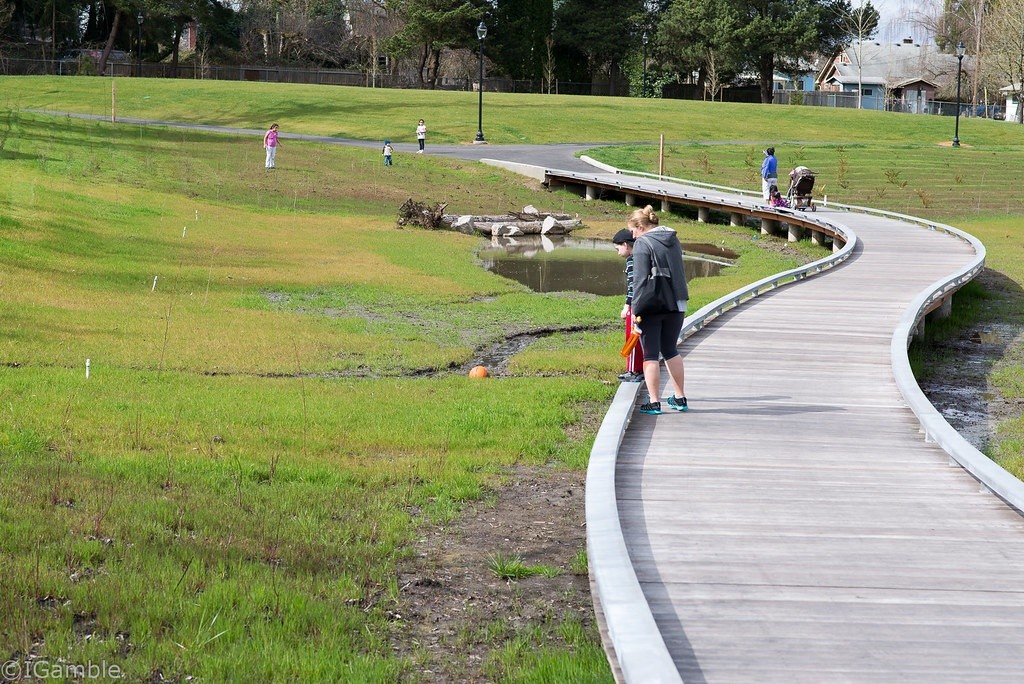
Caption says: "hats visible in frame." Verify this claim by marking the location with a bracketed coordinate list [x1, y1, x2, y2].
[384, 140, 390, 144]
[613, 228, 636, 243]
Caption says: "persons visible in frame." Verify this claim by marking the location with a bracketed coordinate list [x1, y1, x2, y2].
[612, 228, 644, 382]
[263, 122, 282, 168]
[626, 205, 691, 414]
[415, 118, 427, 154]
[761, 148, 778, 203]
[770, 186, 789, 208]
[382, 140, 393, 166]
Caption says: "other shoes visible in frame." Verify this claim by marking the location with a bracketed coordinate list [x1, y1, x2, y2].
[416, 150, 424, 154]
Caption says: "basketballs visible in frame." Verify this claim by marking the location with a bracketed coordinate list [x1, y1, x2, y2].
[468, 365, 491, 379]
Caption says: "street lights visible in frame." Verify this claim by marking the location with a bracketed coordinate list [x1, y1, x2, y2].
[136, 11, 145, 75]
[952, 41, 967, 146]
[475, 22, 488, 142]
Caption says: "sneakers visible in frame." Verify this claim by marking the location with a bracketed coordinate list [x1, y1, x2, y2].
[640, 401, 662, 414]
[666, 395, 689, 410]
[618, 369, 645, 382]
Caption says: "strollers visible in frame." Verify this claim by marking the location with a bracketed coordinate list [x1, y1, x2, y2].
[784, 166, 821, 212]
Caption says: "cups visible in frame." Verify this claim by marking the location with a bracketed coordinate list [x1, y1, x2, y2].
[620, 324, 642, 358]
